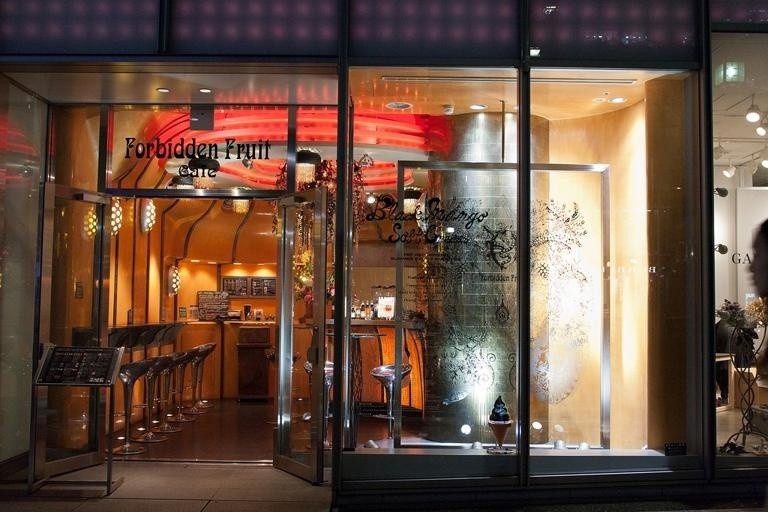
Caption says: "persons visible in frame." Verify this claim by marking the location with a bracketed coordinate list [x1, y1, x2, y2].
[749, 216, 768, 386]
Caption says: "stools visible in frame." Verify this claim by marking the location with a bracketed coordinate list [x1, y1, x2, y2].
[296, 354, 412, 450]
[72, 324, 218, 456]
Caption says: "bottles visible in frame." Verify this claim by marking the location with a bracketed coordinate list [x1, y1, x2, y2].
[351, 299, 374, 320]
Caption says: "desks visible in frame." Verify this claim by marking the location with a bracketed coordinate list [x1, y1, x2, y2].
[172, 322, 432, 400]
[713, 352, 736, 411]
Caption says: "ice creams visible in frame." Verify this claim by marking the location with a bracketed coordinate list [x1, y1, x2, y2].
[487, 395, 512, 449]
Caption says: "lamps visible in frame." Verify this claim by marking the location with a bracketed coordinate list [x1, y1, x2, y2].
[140, 198, 157, 234]
[712, 43, 768, 254]
[111, 200, 123, 235]
[168, 266, 182, 296]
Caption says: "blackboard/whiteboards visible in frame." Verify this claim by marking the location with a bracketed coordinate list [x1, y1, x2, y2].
[197, 291, 229, 321]
